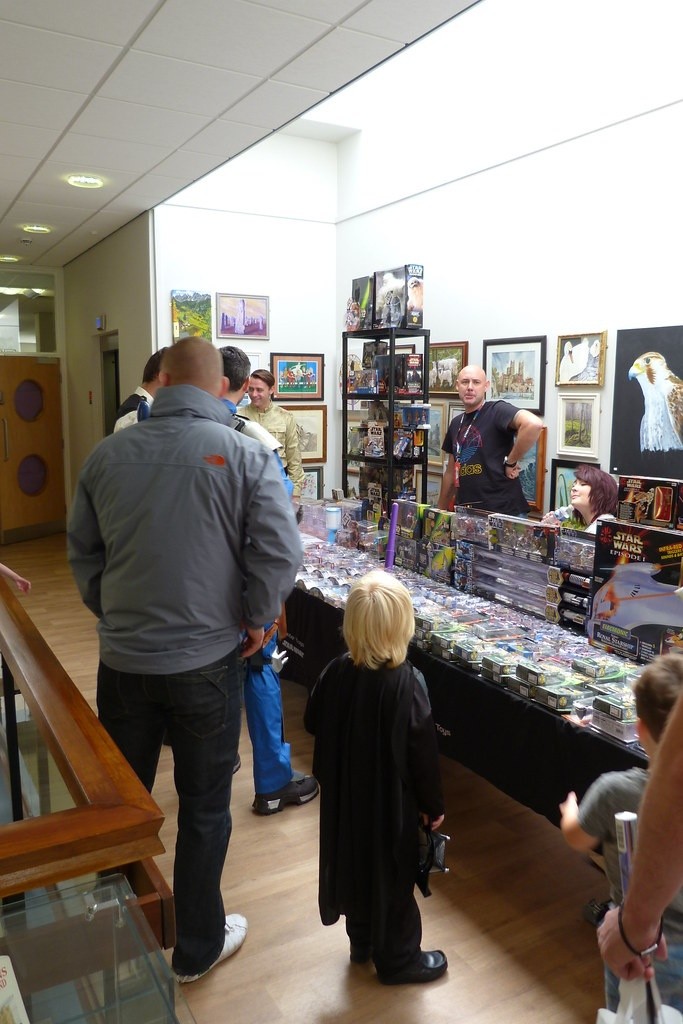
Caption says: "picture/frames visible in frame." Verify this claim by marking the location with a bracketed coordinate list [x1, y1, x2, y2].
[415, 469, 443, 507]
[235, 351, 265, 407]
[514, 426, 544, 514]
[553, 330, 608, 387]
[345, 417, 361, 474]
[416, 401, 447, 467]
[557, 392, 601, 460]
[277, 405, 327, 463]
[386, 344, 415, 355]
[270, 352, 325, 401]
[301, 466, 324, 500]
[483, 335, 547, 416]
[550, 459, 600, 511]
[428, 340, 469, 399]
[216, 292, 270, 341]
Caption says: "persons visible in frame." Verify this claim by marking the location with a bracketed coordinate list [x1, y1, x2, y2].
[598, 690, 683, 983]
[113, 346, 169, 433]
[559, 653, 683, 1015]
[67, 336, 304, 983]
[302, 573, 448, 985]
[437, 365, 544, 520]
[219, 346, 319, 814]
[0, 563, 31, 592]
[544, 464, 618, 534]
[237, 368, 304, 499]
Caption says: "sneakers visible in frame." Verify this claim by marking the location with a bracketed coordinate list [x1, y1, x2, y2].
[252, 777, 319, 815]
[232, 752, 241, 775]
[172, 913, 248, 983]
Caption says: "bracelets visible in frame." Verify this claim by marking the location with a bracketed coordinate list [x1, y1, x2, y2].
[618, 903, 664, 956]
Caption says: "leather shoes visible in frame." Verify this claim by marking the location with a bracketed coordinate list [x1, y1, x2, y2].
[349, 939, 373, 964]
[378, 950, 447, 986]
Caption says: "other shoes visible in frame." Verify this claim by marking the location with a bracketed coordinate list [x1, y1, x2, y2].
[583, 899, 610, 927]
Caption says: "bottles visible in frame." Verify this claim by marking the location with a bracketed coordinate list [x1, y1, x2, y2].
[541, 504, 574, 525]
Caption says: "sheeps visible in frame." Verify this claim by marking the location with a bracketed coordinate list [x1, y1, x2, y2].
[429, 361, 438, 387]
[436, 359, 458, 387]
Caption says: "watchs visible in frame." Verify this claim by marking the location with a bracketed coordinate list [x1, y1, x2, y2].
[503, 456, 517, 467]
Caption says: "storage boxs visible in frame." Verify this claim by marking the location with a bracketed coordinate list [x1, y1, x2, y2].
[373, 264, 423, 329]
[349, 350, 683, 744]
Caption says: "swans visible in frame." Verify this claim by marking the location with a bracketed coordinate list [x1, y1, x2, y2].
[559, 338, 589, 382]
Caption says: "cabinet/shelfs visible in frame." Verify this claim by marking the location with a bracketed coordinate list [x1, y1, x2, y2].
[341, 327, 431, 518]
